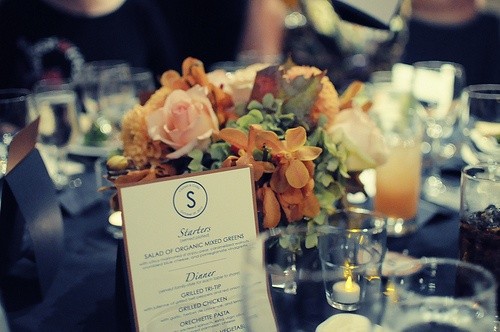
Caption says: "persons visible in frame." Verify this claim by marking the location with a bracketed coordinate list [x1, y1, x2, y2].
[1, 0, 500, 142]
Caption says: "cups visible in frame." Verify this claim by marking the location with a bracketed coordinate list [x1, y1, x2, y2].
[316, 208, 388, 310]
[81, 60, 154, 141]
[388, 256, 496, 332]
[460, 163, 500, 250]
[0, 87, 38, 169]
[370, 117, 420, 226]
[244, 225, 386, 331]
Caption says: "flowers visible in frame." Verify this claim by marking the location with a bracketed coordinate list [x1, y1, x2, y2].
[96, 57, 389, 255]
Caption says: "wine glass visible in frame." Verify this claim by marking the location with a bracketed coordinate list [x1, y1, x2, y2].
[34, 78, 86, 174]
[461, 84, 500, 178]
[413, 59, 465, 204]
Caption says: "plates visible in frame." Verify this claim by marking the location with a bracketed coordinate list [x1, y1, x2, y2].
[68, 134, 121, 157]
